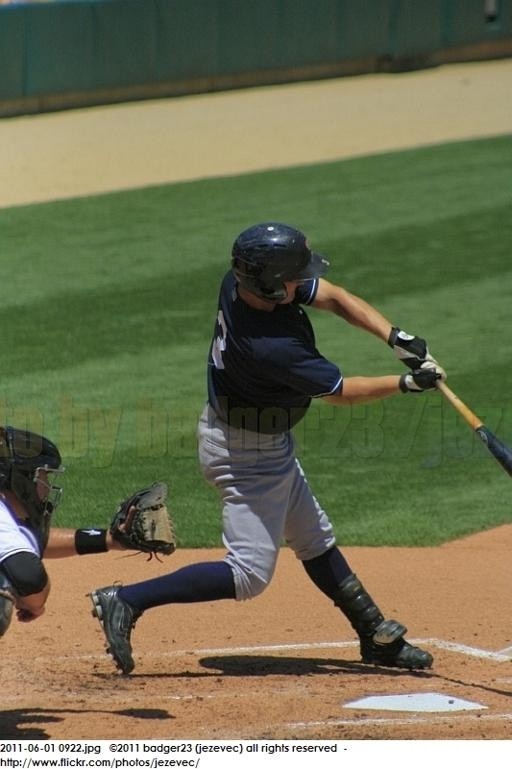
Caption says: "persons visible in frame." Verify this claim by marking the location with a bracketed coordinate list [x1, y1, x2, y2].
[85, 221, 451, 672]
[0, 425, 175, 643]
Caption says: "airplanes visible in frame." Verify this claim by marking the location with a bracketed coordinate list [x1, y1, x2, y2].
[110, 480, 177, 554]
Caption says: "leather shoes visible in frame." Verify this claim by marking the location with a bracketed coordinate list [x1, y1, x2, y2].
[389, 326, 439, 369]
[400, 362, 447, 393]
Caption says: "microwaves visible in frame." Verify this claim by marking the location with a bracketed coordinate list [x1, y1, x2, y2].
[436, 382, 512, 473]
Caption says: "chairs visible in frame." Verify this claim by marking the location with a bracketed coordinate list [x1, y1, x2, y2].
[359, 642, 433, 669]
[90, 585, 144, 675]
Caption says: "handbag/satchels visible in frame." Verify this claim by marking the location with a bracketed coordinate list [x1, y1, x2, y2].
[73, 527, 107, 556]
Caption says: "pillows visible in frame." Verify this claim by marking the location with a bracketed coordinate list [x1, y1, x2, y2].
[1, 426, 65, 560]
[230, 222, 332, 304]
